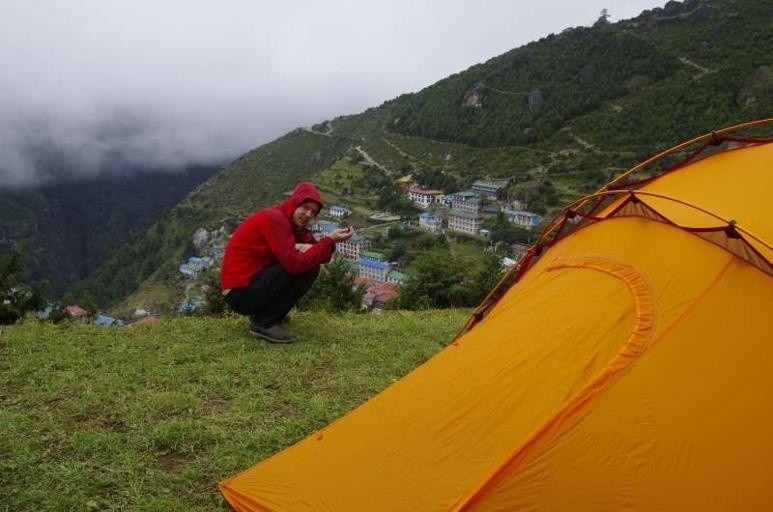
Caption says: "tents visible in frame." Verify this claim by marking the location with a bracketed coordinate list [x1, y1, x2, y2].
[209, 119, 769, 511]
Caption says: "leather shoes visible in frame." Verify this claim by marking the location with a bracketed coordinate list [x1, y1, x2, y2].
[249, 323, 297, 342]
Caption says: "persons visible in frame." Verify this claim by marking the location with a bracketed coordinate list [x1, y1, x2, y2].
[218, 182, 354, 344]
